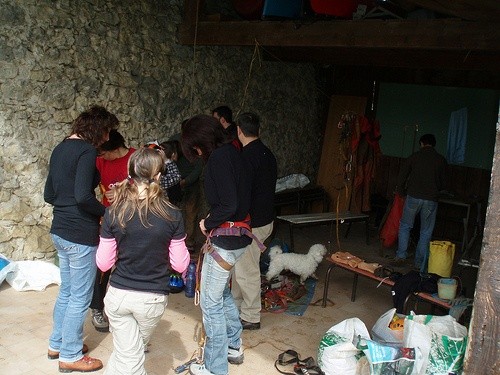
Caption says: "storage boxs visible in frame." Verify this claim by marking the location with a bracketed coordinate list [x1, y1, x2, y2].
[260, 0, 301, 21]
[437, 278, 459, 300]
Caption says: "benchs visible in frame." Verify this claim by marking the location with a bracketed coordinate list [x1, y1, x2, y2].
[311, 255, 468, 316]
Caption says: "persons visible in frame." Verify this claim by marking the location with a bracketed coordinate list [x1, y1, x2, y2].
[43, 103, 120, 372]
[93, 105, 277, 375]
[391, 134, 447, 269]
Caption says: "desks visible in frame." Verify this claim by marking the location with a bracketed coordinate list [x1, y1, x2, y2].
[376, 191, 474, 253]
[274, 171, 330, 214]
[276, 210, 371, 253]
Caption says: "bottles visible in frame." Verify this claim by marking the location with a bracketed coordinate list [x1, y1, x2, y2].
[185, 260, 196, 297]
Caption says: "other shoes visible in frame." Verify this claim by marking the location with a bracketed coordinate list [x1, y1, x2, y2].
[91, 310, 109, 332]
[48, 343, 88, 360]
[58, 355, 103, 373]
[239, 318, 260, 330]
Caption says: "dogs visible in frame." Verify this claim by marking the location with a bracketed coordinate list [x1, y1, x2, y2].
[267, 243, 328, 286]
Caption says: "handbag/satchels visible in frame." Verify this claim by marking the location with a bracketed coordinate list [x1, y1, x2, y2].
[427, 240, 455, 278]
[317, 308, 468, 375]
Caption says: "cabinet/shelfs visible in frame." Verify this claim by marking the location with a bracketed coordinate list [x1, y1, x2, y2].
[310, 95, 377, 214]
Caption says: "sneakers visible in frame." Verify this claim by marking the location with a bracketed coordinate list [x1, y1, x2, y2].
[189, 363, 230, 375]
[228, 338, 244, 364]
